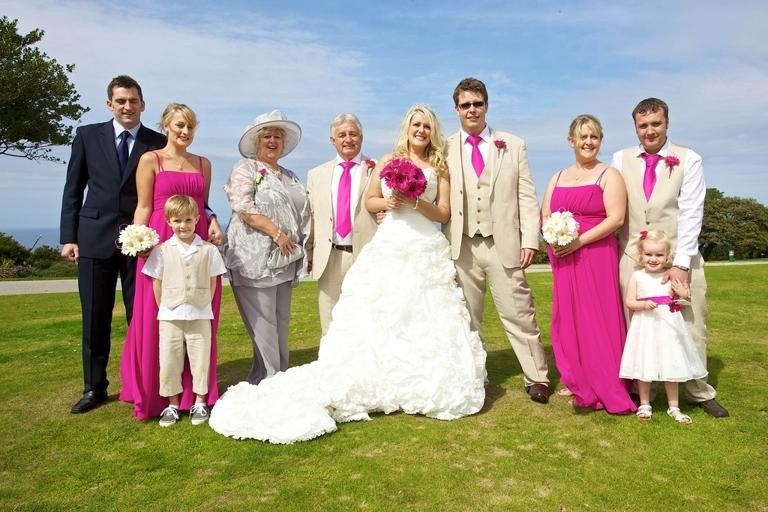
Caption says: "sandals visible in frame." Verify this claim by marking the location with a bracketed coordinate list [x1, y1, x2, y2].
[636, 405, 652, 420]
[666, 407, 692, 424]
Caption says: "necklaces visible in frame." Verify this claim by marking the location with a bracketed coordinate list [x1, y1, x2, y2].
[174, 152, 190, 170]
[573, 166, 591, 180]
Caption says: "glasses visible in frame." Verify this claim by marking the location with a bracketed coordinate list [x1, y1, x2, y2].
[461, 101, 484, 109]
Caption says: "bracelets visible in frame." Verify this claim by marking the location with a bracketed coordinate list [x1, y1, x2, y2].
[674, 264, 689, 272]
[412, 196, 420, 210]
[273, 227, 282, 242]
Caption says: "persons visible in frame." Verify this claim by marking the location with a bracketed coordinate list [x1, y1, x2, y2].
[303, 110, 382, 340]
[120, 103, 221, 423]
[140, 195, 226, 428]
[317, 103, 487, 420]
[609, 98, 730, 417]
[226, 110, 305, 386]
[539, 115, 628, 418]
[624, 230, 692, 423]
[443, 78, 552, 404]
[59, 74, 167, 415]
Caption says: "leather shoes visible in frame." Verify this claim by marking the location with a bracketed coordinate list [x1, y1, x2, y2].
[524, 384, 548, 404]
[70, 388, 107, 414]
[699, 399, 729, 417]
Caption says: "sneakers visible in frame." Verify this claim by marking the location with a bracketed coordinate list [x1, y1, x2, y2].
[159, 407, 179, 426]
[190, 405, 209, 426]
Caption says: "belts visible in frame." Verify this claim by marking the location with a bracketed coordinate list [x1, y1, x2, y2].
[332, 243, 352, 252]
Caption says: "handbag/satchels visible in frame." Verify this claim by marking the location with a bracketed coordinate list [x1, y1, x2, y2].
[267, 245, 305, 269]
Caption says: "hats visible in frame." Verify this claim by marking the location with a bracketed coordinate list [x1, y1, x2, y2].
[238, 109, 302, 159]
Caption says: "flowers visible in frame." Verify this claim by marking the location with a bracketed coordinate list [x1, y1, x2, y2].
[379, 158, 427, 197]
[658, 297, 687, 313]
[493, 139, 506, 154]
[666, 153, 679, 176]
[256, 168, 266, 185]
[541, 210, 578, 247]
[116, 224, 160, 257]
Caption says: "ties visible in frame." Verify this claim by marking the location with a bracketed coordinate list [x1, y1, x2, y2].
[117, 131, 130, 178]
[466, 135, 484, 177]
[640, 153, 661, 202]
[335, 161, 356, 238]
